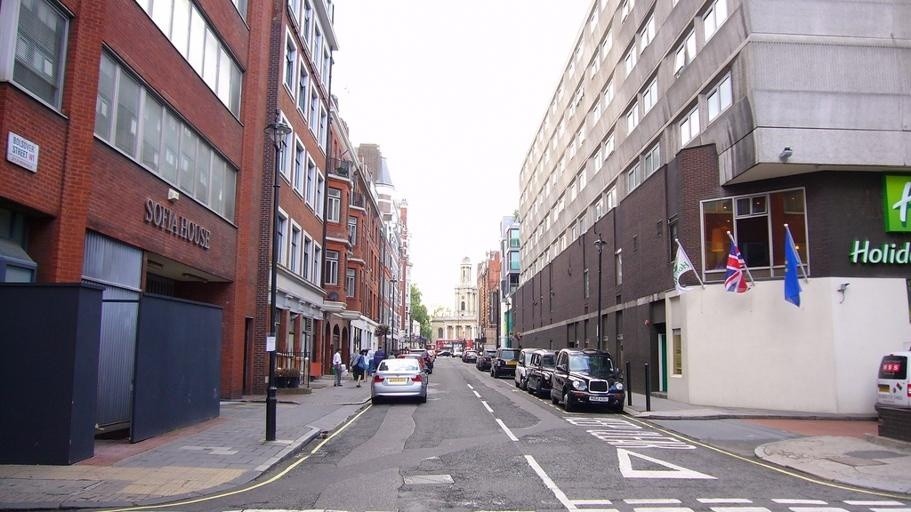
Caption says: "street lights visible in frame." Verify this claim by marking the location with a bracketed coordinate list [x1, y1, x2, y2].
[389, 275, 398, 356]
[594, 233, 607, 351]
[264, 107, 290, 443]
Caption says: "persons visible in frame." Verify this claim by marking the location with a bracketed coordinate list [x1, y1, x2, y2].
[332, 348, 343, 386]
[352, 346, 395, 387]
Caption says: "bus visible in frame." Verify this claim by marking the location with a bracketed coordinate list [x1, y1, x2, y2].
[424, 344, 436, 356]
[435, 338, 474, 350]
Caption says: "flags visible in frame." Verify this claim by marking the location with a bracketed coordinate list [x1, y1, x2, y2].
[725, 240, 752, 294]
[783, 227, 803, 307]
[672, 246, 692, 290]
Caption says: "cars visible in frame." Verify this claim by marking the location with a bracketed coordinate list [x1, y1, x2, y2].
[490, 347, 520, 379]
[438, 348, 497, 372]
[371, 358, 428, 405]
[526, 348, 561, 399]
[874, 350, 911, 411]
[550, 347, 625, 413]
[397, 349, 437, 374]
[515, 347, 541, 390]
